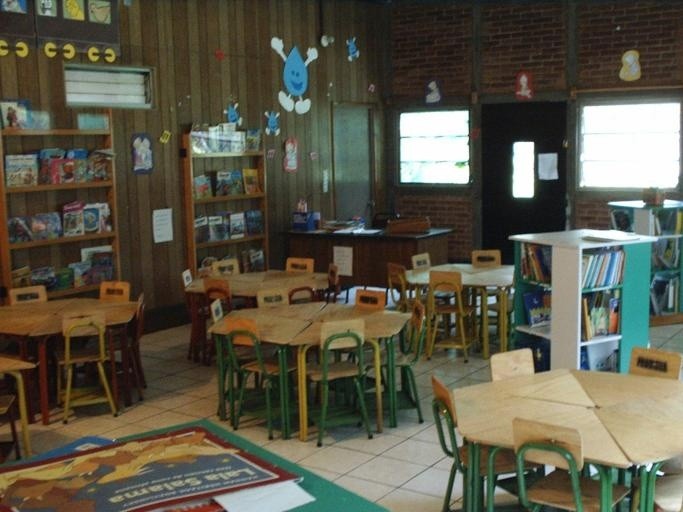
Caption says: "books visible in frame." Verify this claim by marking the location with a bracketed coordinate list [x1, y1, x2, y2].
[0, 98, 115, 295]
[611, 209, 683, 315]
[190, 121, 266, 277]
[522, 242, 625, 341]
[0, 427, 306, 512]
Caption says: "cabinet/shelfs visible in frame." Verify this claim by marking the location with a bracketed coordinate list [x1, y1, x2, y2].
[0, 106, 123, 299]
[507, 227, 656, 372]
[181, 133, 268, 278]
[608, 197, 682, 325]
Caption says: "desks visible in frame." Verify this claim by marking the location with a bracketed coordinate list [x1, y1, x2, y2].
[0, 419, 391, 512]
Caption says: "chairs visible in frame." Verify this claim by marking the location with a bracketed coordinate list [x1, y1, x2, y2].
[432, 349, 683, 512]
[2, 282, 146, 459]
[387, 249, 515, 364]
[370, 212, 401, 228]
[182, 257, 424, 447]
